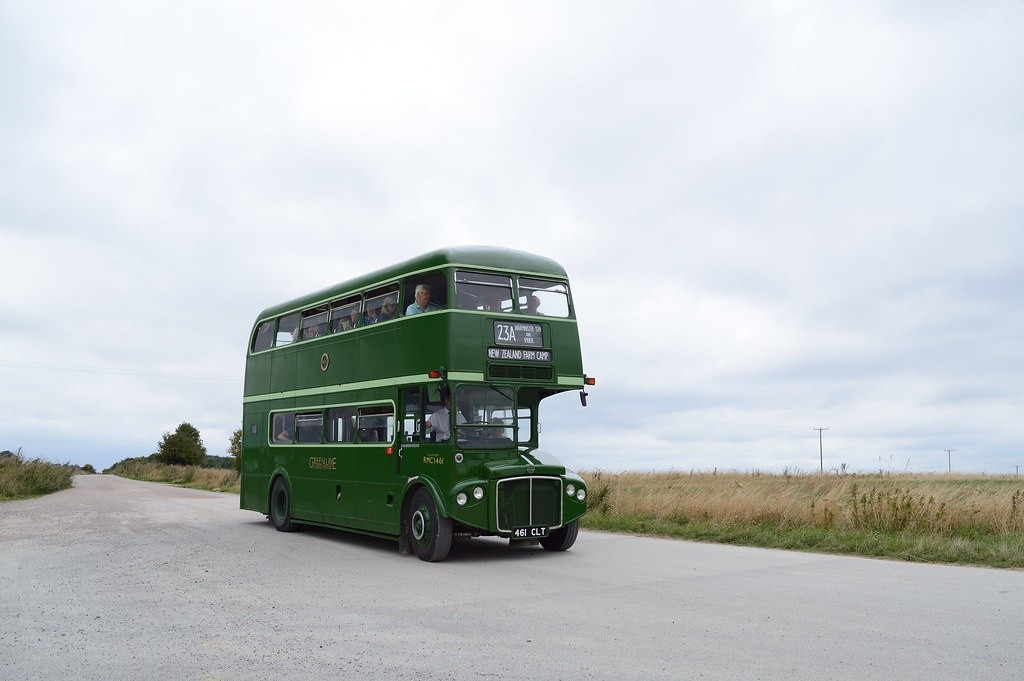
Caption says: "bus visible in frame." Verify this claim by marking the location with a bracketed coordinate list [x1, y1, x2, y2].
[239, 245, 596, 564]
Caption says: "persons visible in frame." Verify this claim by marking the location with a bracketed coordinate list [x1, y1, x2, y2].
[488, 419, 511, 441]
[423, 389, 468, 443]
[519, 296, 544, 316]
[290, 295, 398, 343]
[350, 417, 380, 443]
[277, 418, 317, 446]
[405, 285, 441, 316]
[488, 295, 505, 314]
